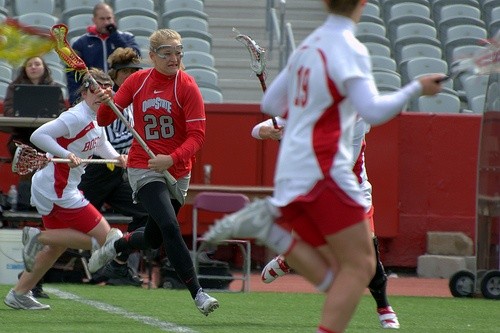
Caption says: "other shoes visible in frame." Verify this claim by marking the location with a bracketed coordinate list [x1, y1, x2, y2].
[18, 271, 49, 299]
[114, 238, 126, 253]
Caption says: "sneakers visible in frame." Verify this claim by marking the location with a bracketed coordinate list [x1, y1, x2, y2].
[261, 255, 293, 284]
[88, 228, 123, 273]
[377, 306, 400, 329]
[194, 288, 219, 316]
[89, 261, 118, 284]
[103, 260, 143, 287]
[204, 200, 273, 246]
[4, 288, 50, 310]
[22, 226, 44, 272]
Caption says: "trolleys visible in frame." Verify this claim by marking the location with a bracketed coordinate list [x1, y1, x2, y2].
[449, 60, 498, 296]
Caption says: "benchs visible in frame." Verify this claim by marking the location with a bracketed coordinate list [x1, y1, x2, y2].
[0, 157, 153, 288]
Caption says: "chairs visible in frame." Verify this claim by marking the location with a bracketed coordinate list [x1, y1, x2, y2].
[0, 0, 221, 102]
[193, 192, 251, 293]
[354, 0, 500, 114]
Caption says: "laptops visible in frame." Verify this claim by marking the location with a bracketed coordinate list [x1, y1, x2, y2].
[13, 84, 59, 118]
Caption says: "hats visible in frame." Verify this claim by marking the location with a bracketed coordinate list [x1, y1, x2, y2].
[111, 58, 150, 69]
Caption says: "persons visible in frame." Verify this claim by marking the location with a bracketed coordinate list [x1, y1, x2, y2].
[3, 68, 128, 309]
[251, 115, 400, 331]
[17, 47, 142, 299]
[88, 29, 219, 317]
[203, 1, 447, 333]
[3, 56, 65, 213]
[67, 3, 140, 108]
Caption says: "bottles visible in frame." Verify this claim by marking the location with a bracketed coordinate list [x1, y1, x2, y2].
[204, 164, 212, 184]
[7, 184, 18, 211]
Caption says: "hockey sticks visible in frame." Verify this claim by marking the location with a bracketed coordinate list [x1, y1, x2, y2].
[432, 35, 500, 85]
[9, 137, 123, 177]
[236, 32, 282, 144]
[50, 24, 178, 187]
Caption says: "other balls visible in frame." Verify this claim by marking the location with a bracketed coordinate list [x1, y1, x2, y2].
[59, 47, 70, 58]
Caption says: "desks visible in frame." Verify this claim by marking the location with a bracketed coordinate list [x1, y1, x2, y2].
[181, 186, 275, 264]
